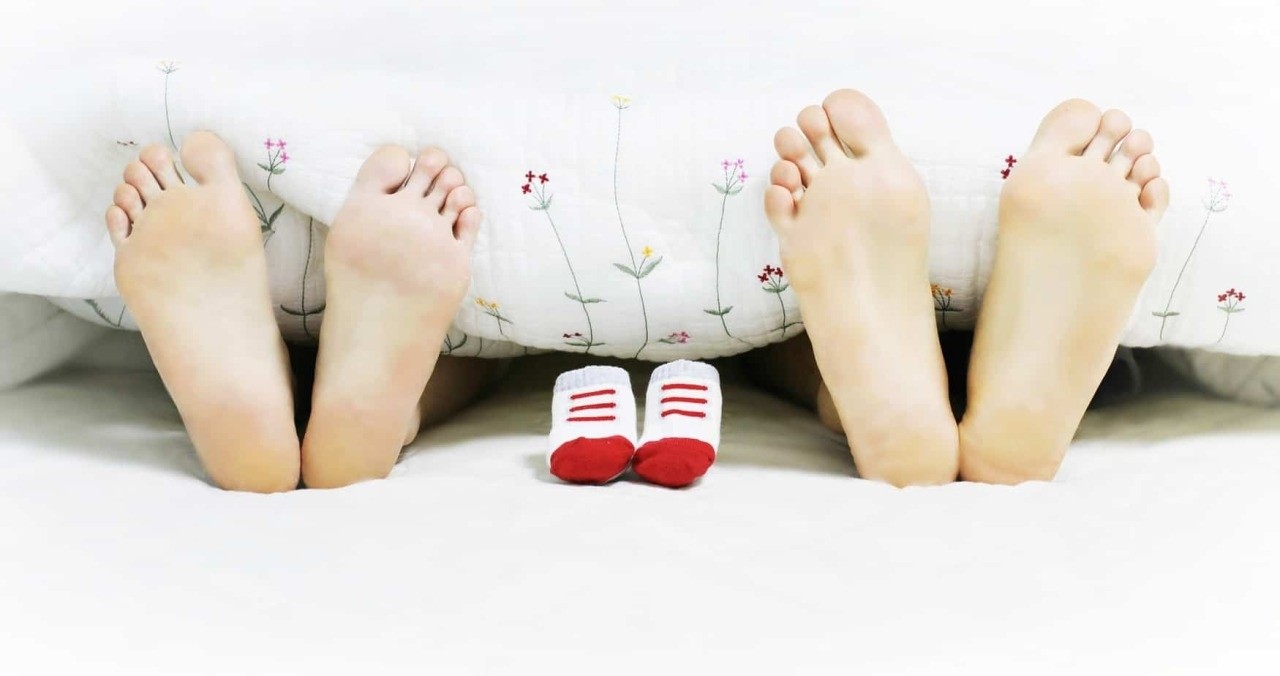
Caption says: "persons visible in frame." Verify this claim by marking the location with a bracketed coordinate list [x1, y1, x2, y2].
[106, 132, 495, 493]
[764, 87, 1169, 490]
